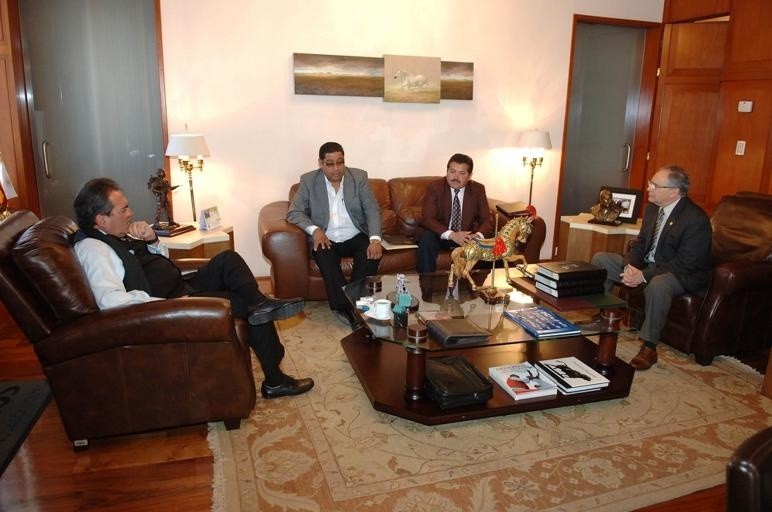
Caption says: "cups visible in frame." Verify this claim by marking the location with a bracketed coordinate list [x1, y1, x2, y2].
[373, 299, 392, 318]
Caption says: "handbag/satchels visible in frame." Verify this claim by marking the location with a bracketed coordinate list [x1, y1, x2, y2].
[424, 353, 494, 410]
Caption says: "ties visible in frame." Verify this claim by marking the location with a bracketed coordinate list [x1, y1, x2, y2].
[644, 207, 664, 263]
[451, 189, 462, 232]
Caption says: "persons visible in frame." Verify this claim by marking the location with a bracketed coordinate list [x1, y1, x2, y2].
[69, 176, 315, 400]
[588, 162, 713, 372]
[591, 189, 622, 223]
[417, 152, 493, 274]
[284, 141, 383, 316]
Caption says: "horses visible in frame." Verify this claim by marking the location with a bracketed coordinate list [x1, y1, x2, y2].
[447, 215, 534, 291]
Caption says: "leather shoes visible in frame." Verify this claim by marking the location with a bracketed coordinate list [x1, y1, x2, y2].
[345, 306, 366, 331]
[629, 345, 657, 370]
[260, 376, 314, 399]
[574, 314, 600, 331]
[247, 296, 306, 325]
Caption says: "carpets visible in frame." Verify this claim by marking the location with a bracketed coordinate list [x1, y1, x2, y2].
[205, 269, 772, 511]
[0, 379, 53, 477]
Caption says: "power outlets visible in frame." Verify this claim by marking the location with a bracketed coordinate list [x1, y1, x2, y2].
[735, 141, 746, 156]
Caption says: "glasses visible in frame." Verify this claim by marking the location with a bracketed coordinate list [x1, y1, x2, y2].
[322, 159, 344, 167]
[647, 177, 680, 190]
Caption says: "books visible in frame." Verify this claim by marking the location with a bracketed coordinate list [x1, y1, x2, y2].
[515, 258, 608, 299]
[534, 355, 611, 395]
[496, 201, 532, 217]
[382, 233, 420, 251]
[503, 305, 582, 339]
[488, 360, 557, 402]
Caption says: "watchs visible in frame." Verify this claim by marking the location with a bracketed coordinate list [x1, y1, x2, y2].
[370, 238, 382, 245]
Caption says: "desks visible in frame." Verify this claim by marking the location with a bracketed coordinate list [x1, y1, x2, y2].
[340, 267, 636, 426]
[555, 213, 642, 264]
[149, 221, 235, 273]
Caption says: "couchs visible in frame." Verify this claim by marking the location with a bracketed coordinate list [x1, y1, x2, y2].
[258, 176, 546, 301]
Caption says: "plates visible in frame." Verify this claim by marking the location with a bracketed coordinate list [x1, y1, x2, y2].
[364, 310, 394, 320]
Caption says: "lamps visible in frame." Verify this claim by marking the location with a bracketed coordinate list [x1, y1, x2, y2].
[515, 128, 553, 206]
[164, 124, 211, 222]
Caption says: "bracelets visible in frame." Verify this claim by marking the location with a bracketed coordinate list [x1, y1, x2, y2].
[146, 231, 158, 245]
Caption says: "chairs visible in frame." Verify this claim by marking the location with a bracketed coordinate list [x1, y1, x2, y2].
[725, 426, 772, 511]
[0, 210, 257, 452]
[619, 191, 772, 366]
